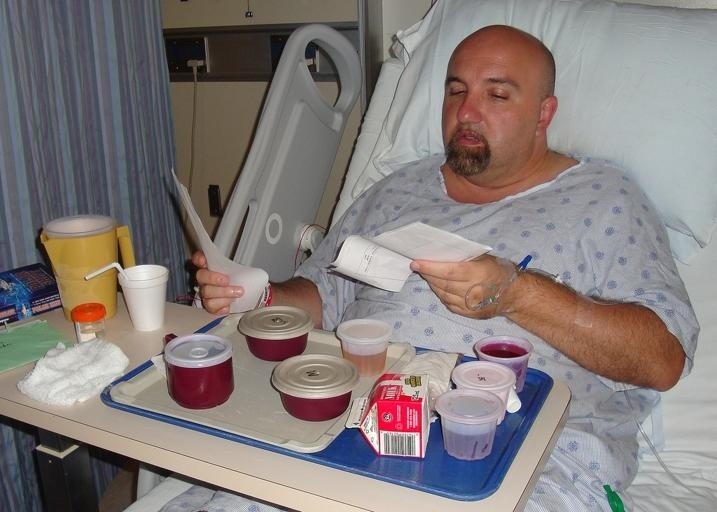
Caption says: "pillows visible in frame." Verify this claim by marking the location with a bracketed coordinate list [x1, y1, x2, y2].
[352, 0, 716, 267]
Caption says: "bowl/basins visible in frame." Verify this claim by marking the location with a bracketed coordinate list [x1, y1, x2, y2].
[271, 353, 360, 422]
[237, 305, 316, 362]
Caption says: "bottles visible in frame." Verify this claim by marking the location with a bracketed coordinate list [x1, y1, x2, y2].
[70, 302, 106, 344]
[162, 333, 235, 411]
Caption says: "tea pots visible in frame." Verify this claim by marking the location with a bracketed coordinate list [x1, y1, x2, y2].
[39, 213, 137, 324]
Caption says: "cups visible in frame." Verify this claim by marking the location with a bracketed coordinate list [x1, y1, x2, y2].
[117, 264, 170, 332]
[473, 334, 535, 394]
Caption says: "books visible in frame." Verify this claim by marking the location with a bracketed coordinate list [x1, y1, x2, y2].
[0, 262, 63, 328]
